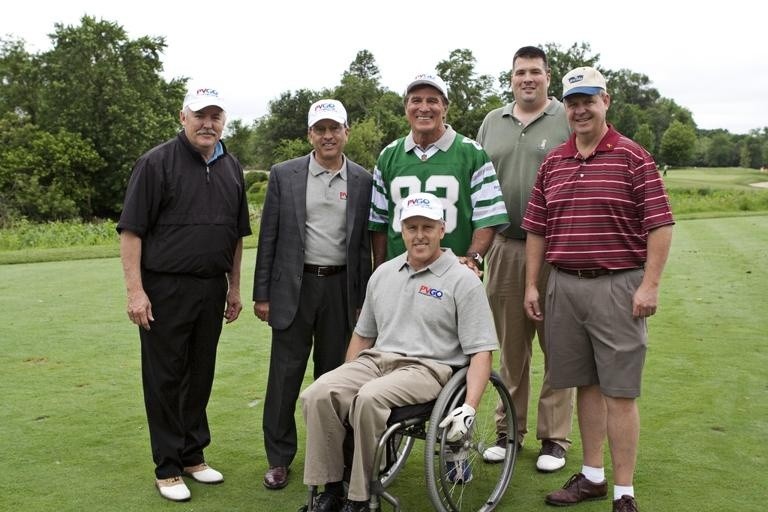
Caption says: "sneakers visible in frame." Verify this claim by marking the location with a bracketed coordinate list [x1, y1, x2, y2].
[444, 445, 472, 484]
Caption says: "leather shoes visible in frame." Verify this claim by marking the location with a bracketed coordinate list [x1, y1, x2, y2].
[181, 463, 225, 486]
[261, 465, 291, 490]
[297, 491, 346, 511]
[536, 440, 565, 472]
[544, 472, 608, 507]
[483, 433, 522, 464]
[337, 499, 371, 512]
[156, 475, 191, 502]
[612, 494, 637, 512]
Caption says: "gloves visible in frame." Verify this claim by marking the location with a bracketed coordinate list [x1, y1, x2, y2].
[438, 402, 477, 442]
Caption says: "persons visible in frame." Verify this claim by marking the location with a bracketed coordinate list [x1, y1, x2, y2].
[474, 47, 577, 474]
[520, 66, 673, 512]
[297, 192, 500, 512]
[253, 99, 374, 490]
[368, 72, 511, 276]
[117, 85, 253, 501]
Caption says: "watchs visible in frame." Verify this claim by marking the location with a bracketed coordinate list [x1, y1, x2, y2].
[465, 251, 483, 267]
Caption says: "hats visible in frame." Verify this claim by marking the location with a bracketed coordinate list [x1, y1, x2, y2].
[399, 192, 444, 222]
[182, 83, 226, 113]
[307, 98, 348, 129]
[561, 66, 607, 101]
[404, 73, 449, 102]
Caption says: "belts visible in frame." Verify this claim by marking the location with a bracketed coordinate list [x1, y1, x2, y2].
[301, 263, 348, 277]
[143, 269, 227, 281]
[553, 264, 644, 280]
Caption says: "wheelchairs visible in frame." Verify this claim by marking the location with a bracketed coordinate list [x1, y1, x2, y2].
[296, 365, 517, 512]
